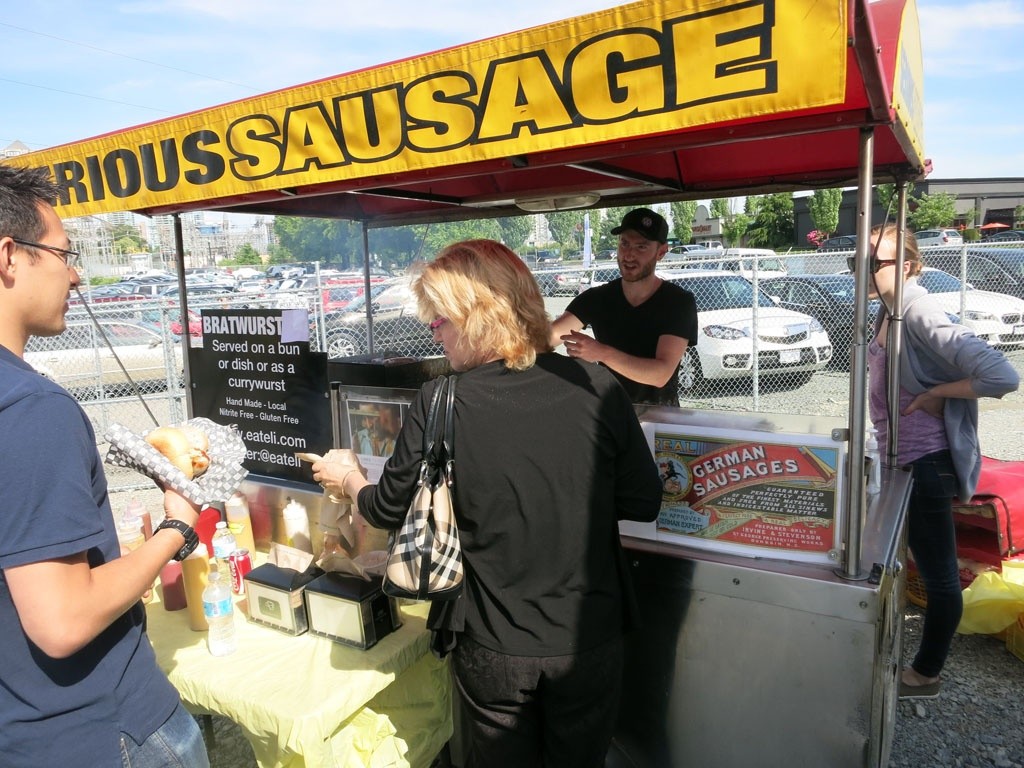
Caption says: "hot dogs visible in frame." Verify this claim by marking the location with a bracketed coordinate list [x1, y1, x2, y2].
[144, 425, 211, 482]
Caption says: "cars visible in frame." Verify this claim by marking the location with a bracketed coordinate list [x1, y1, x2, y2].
[25, 228, 1024, 390]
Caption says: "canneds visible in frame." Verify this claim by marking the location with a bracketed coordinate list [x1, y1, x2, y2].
[228, 546, 251, 595]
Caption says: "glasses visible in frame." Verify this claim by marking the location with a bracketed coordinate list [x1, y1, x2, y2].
[14, 237, 82, 270]
[846, 255, 897, 274]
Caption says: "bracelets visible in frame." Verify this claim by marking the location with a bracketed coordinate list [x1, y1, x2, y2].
[342, 468, 359, 499]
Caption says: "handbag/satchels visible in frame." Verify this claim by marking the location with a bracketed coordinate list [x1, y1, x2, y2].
[378, 373, 467, 604]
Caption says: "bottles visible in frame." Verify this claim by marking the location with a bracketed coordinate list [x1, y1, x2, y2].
[159, 503, 221, 630]
[212, 522, 237, 587]
[225, 490, 256, 561]
[283, 498, 314, 555]
[202, 571, 237, 658]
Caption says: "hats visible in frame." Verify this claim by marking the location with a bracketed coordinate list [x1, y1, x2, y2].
[609, 208, 669, 245]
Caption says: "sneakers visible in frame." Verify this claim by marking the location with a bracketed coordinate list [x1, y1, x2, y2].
[898, 674, 941, 701]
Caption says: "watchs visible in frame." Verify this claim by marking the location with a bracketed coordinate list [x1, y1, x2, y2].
[151, 519, 199, 563]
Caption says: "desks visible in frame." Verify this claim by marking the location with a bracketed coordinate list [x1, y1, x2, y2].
[113, 513, 451, 767]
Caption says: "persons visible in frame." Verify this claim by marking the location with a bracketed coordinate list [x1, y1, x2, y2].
[545, 205, 699, 412]
[851, 223, 1019, 702]
[0, 165, 213, 768]
[309, 236, 665, 768]
